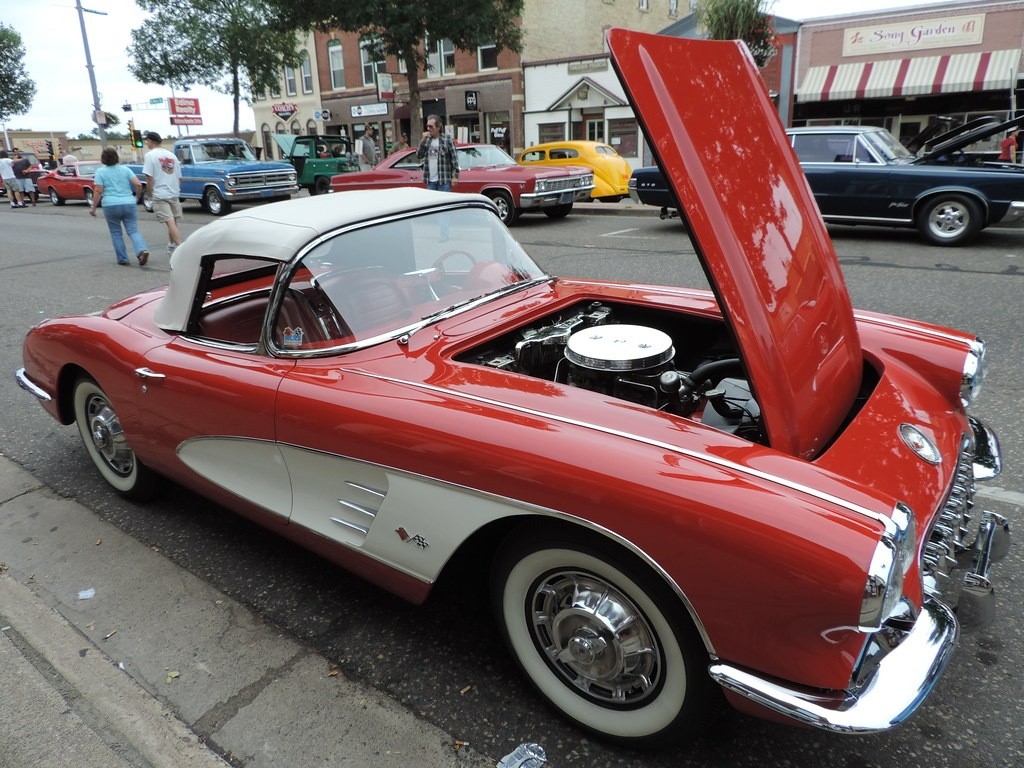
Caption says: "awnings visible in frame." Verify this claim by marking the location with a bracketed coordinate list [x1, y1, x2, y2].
[798, 48, 1022, 103]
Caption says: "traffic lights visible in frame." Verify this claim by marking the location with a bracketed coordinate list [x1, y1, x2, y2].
[132, 129, 143, 148]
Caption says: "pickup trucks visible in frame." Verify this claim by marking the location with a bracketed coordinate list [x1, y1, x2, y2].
[123, 137, 299, 216]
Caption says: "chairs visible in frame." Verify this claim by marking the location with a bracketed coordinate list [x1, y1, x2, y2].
[310, 264, 415, 339]
[194, 286, 333, 348]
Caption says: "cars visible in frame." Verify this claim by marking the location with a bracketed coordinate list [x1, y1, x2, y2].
[627, 112, 1024, 244]
[34, 161, 103, 208]
[15, 29, 1011, 756]
[329, 141, 595, 227]
[514, 141, 633, 202]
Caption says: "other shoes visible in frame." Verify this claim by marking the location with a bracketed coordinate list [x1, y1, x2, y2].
[138, 250, 149, 266]
[166, 246, 175, 255]
[33, 205, 36, 206]
[18, 205, 26, 208]
[12, 205, 18, 208]
[22, 203, 28, 207]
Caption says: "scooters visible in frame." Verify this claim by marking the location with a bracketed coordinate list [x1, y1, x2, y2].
[0, 151, 50, 203]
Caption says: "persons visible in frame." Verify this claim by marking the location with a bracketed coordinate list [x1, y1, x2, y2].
[10, 149, 36, 206]
[418, 116, 461, 242]
[143, 131, 185, 255]
[387, 134, 412, 156]
[0, 149, 27, 208]
[90, 147, 150, 266]
[998, 126, 1020, 163]
[355, 123, 378, 173]
